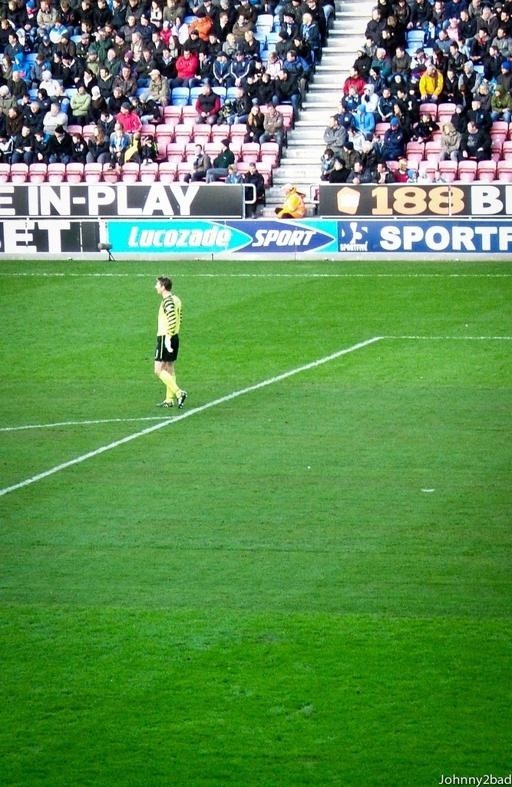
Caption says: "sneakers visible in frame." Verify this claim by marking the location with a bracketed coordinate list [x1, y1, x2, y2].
[156, 401, 174, 408]
[176, 390, 187, 408]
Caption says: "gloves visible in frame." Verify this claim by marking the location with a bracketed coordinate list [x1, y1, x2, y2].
[165, 335, 173, 352]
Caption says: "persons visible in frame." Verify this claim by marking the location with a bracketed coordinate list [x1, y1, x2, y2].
[0, 1, 510, 218]
[150, 275, 189, 412]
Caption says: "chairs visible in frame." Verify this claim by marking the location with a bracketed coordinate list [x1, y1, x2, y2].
[375, 103, 511, 181]
[406, 30, 425, 49]
[0, 13, 294, 207]
